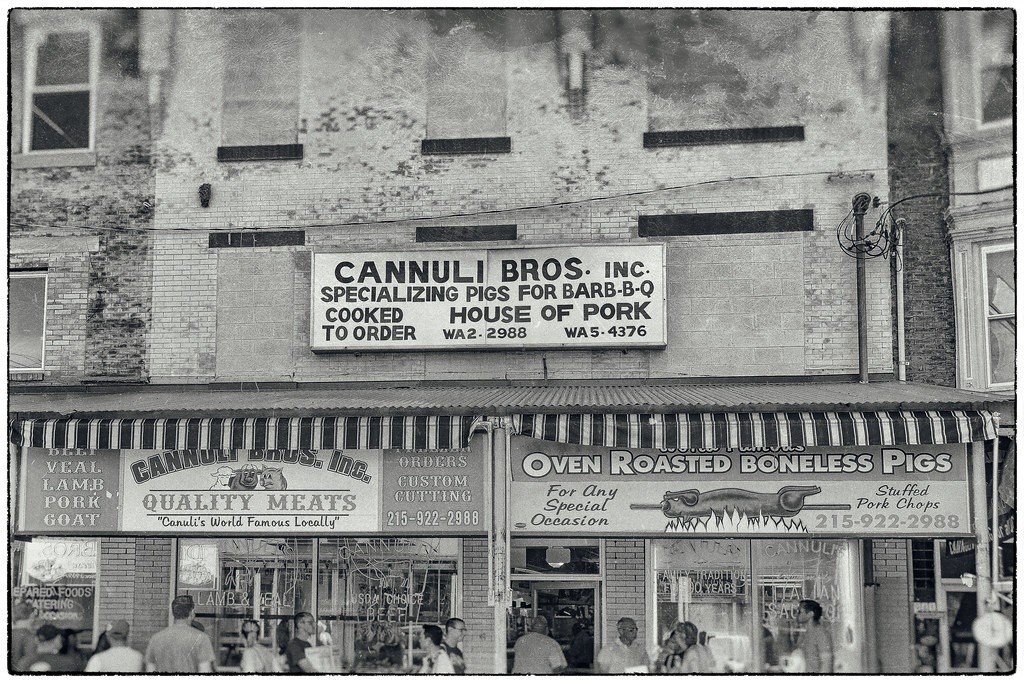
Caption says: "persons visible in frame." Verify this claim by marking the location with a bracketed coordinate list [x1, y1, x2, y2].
[239, 619, 281, 673]
[512, 616, 568, 674]
[653, 620, 712, 674]
[419, 618, 467, 674]
[596, 617, 650, 674]
[11, 594, 214, 673]
[284, 612, 322, 673]
[795, 600, 833, 673]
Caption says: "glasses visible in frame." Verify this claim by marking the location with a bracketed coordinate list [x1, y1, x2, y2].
[450, 626, 467, 632]
[618, 627, 639, 633]
[797, 609, 810, 615]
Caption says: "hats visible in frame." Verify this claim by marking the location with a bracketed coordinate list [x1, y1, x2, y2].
[105, 620, 130, 638]
[36, 624, 59, 641]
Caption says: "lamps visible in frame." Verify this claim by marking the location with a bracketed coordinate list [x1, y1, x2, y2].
[546, 546, 571, 569]
